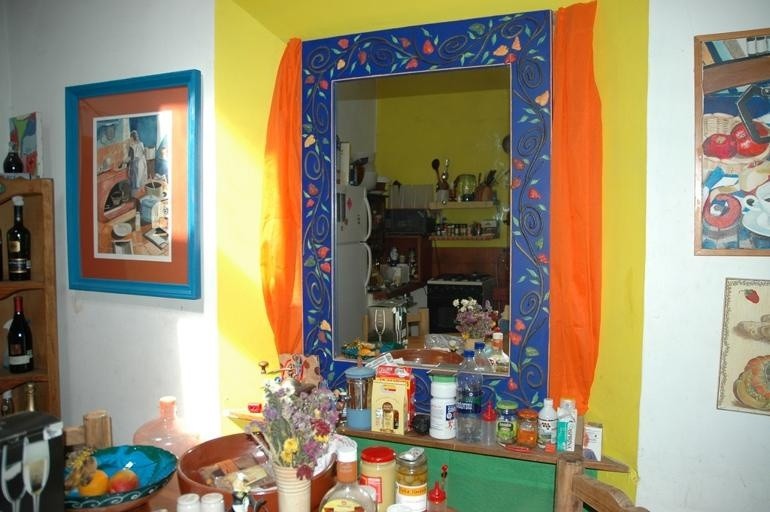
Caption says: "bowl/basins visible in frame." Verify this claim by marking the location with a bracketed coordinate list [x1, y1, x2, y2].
[178, 434, 335, 512]
[380, 349, 460, 366]
[340, 344, 380, 359]
[66, 443, 176, 511]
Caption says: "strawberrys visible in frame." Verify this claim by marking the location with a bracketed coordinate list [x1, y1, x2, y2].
[745, 290, 759, 303]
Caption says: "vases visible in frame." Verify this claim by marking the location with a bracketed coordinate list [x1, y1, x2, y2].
[271, 461, 312, 512]
[464, 338, 484, 351]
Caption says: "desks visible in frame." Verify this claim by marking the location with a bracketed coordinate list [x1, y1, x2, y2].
[336, 331, 509, 374]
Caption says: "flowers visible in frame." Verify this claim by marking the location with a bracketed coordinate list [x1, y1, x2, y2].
[246, 377, 340, 480]
[452, 297, 495, 338]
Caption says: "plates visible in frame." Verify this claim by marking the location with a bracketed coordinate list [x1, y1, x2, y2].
[138, 196, 158, 204]
[112, 222, 133, 237]
[742, 209, 770, 238]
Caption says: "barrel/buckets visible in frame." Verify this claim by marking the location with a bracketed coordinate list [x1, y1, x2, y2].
[145, 174, 163, 195]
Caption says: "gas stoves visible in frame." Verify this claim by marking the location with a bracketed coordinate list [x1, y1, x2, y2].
[428, 271, 495, 285]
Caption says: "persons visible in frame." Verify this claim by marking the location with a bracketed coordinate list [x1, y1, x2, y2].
[121, 128, 151, 196]
[97, 124, 118, 149]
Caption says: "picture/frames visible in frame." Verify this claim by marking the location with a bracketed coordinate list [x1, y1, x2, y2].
[65, 69, 201, 300]
[694, 27, 770, 256]
[715, 278, 770, 418]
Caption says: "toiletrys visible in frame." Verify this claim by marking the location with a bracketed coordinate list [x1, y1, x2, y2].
[370, 262, 387, 291]
[397, 254, 411, 285]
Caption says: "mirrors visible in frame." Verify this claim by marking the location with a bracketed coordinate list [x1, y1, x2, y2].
[302, 10, 553, 417]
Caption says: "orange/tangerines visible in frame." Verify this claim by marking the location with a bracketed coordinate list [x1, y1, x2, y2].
[78, 470, 108, 497]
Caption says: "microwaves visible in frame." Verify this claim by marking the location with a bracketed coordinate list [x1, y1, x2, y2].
[368, 299, 411, 343]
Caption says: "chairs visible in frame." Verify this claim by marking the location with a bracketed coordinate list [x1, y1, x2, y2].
[553, 452, 649, 512]
[363, 307, 430, 345]
[66, 411, 112, 452]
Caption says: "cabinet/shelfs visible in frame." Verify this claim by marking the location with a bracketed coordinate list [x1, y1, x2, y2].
[428, 198, 508, 248]
[370, 232, 429, 336]
[0, 178, 60, 428]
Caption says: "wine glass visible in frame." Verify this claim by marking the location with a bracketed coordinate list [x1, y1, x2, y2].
[0, 439, 25, 511]
[22, 432, 51, 511]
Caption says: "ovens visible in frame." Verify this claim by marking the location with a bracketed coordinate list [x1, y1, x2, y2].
[428, 285, 483, 331]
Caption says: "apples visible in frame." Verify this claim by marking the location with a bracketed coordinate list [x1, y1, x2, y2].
[108, 469, 138, 494]
[701, 134, 737, 159]
[729, 121, 769, 156]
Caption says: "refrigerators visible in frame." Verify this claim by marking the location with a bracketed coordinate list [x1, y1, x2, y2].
[333, 183, 374, 355]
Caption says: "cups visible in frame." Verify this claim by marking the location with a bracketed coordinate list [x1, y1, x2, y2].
[436, 190, 449, 205]
[145, 182, 167, 199]
[476, 186, 493, 201]
[359, 484, 379, 503]
[744, 180, 770, 215]
[84, 410, 112, 451]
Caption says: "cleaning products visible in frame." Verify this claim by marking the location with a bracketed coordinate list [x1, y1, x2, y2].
[319, 433, 378, 511]
[537, 397, 558, 450]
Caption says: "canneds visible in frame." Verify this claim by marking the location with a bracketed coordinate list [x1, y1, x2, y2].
[359, 447, 397, 512]
[517, 409, 539, 449]
[395, 451, 428, 512]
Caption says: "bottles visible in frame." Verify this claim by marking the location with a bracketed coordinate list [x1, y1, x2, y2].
[537, 399, 557, 447]
[2, 390, 16, 415]
[3, 131, 24, 174]
[177, 492, 225, 511]
[8, 293, 34, 369]
[346, 366, 373, 431]
[320, 446, 376, 511]
[389, 248, 415, 277]
[23, 382, 38, 412]
[436, 223, 483, 236]
[456, 351, 479, 443]
[460, 342, 493, 373]
[485, 332, 510, 373]
[429, 374, 458, 438]
[7, 198, 32, 280]
[135, 211, 141, 232]
[481, 400, 496, 445]
[425, 480, 446, 511]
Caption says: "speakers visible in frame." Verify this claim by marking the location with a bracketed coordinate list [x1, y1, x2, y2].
[386, 206, 429, 237]
